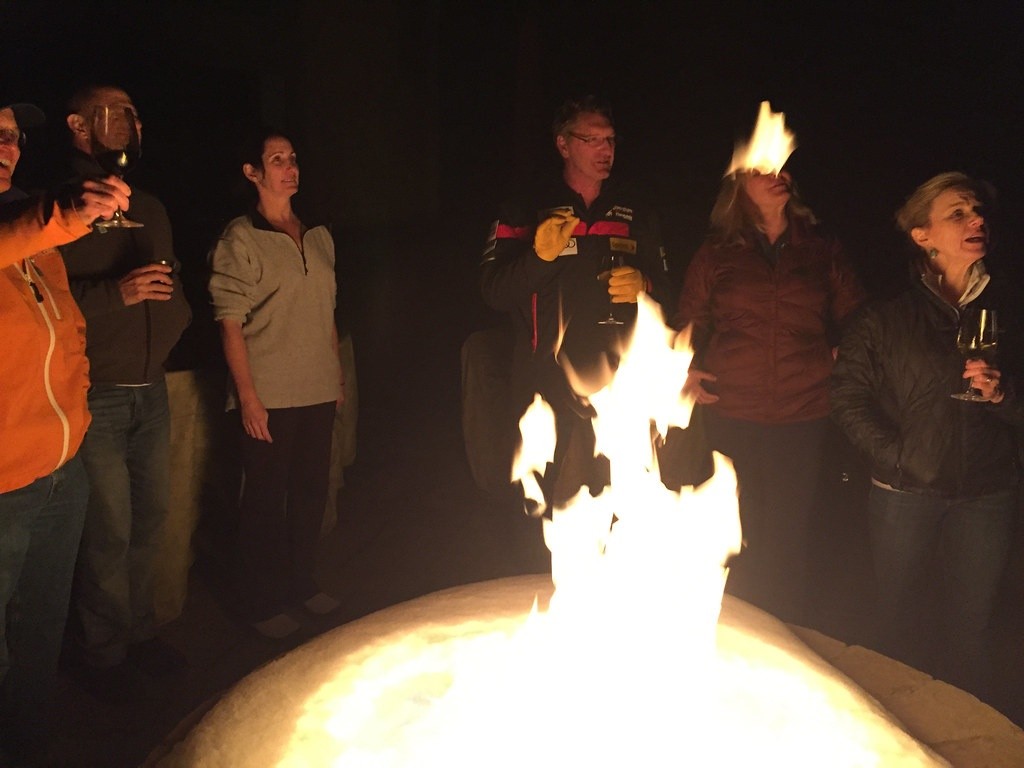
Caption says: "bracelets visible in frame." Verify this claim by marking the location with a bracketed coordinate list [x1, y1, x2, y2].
[339, 382, 345, 386]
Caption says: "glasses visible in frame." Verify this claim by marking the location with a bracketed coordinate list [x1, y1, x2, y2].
[0, 127, 26, 153]
[569, 131, 623, 150]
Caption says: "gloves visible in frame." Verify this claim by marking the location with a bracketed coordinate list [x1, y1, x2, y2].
[597, 266, 647, 304]
[533, 208, 580, 261]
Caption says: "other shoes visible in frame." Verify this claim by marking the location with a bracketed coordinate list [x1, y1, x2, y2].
[303, 592, 343, 615]
[91, 663, 131, 708]
[127, 636, 187, 677]
[250, 613, 300, 641]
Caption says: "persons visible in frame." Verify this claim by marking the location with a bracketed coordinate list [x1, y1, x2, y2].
[478, 93, 868, 625]
[206, 130, 347, 644]
[0, 81, 193, 756]
[828, 172, 1024, 696]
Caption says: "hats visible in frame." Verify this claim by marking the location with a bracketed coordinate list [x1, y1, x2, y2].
[0, 83, 46, 129]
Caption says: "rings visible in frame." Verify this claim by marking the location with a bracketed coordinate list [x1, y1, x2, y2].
[986, 376, 992, 384]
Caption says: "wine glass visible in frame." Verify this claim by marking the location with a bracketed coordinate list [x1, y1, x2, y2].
[951, 308, 999, 402]
[598, 255, 624, 325]
[91, 105, 144, 228]
